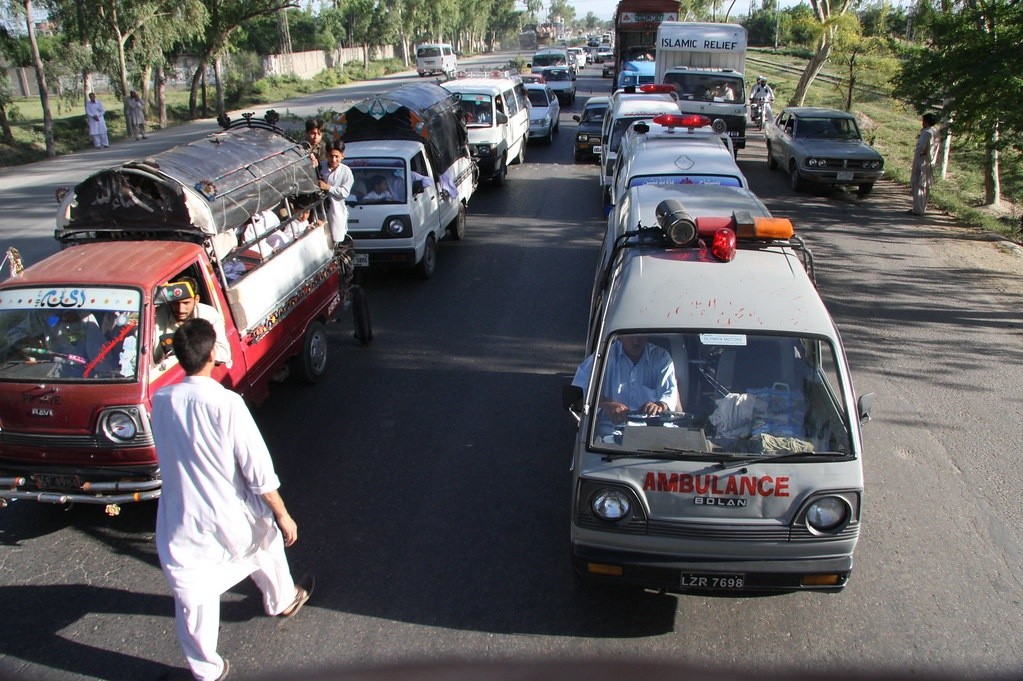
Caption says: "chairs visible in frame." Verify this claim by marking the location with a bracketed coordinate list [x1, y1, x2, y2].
[642, 336, 694, 416]
[713, 336, 805, 438]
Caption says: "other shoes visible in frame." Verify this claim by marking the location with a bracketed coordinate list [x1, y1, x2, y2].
[906, 209, 920, 216]
[142, 136, 148, 139]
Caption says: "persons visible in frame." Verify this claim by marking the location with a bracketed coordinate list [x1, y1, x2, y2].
[906, 113, 940, 215]
[568, 333, 679, 443]
[750, 75, 775, 125]
[5, 275, 234, 374]
[309, 140, 354, 242]
[87, 92, 110, 148]
[477, 102, 503, 123]
[711, 80, 734, 101]
[301, 118, 329, 163]
[153, 318, 316, 681]
[349, 164, 433, 200]
[126, 90, 149, 140]
[240, 193, 323, 254]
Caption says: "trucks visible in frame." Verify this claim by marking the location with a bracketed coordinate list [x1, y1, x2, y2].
[612, 0, 680, 94]
[653, 20, 747, 160]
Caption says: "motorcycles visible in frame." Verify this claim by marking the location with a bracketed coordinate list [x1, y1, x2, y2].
[747, 97, 775, 131]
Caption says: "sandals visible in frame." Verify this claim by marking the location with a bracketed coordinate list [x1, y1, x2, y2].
[283, 572, 316, 618]
[214, 661, 229, 681]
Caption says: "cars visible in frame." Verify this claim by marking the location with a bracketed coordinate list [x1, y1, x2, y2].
[572, 96, 612, 163]
[523, 30, 614, 147]
[764, 106, 886, 193]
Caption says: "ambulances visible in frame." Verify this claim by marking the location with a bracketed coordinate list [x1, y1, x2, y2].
[560, 183, 877, 596]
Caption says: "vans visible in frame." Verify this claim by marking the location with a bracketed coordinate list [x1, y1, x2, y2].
[606, 80, 749, 207]
[439, 72, 530, 185]
[415, 44, 457, 77]
[599, 84, 683, 194]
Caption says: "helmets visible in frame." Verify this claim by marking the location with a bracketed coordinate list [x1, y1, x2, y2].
[760, 77, 767, 84]
[757, 76, 763, 83]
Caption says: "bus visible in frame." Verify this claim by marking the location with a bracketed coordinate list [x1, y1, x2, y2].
[334, 83, 482, 278]
[1, 116, 351, 522]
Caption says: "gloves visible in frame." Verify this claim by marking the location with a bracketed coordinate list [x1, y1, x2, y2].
[158, 333, 177, 356]
